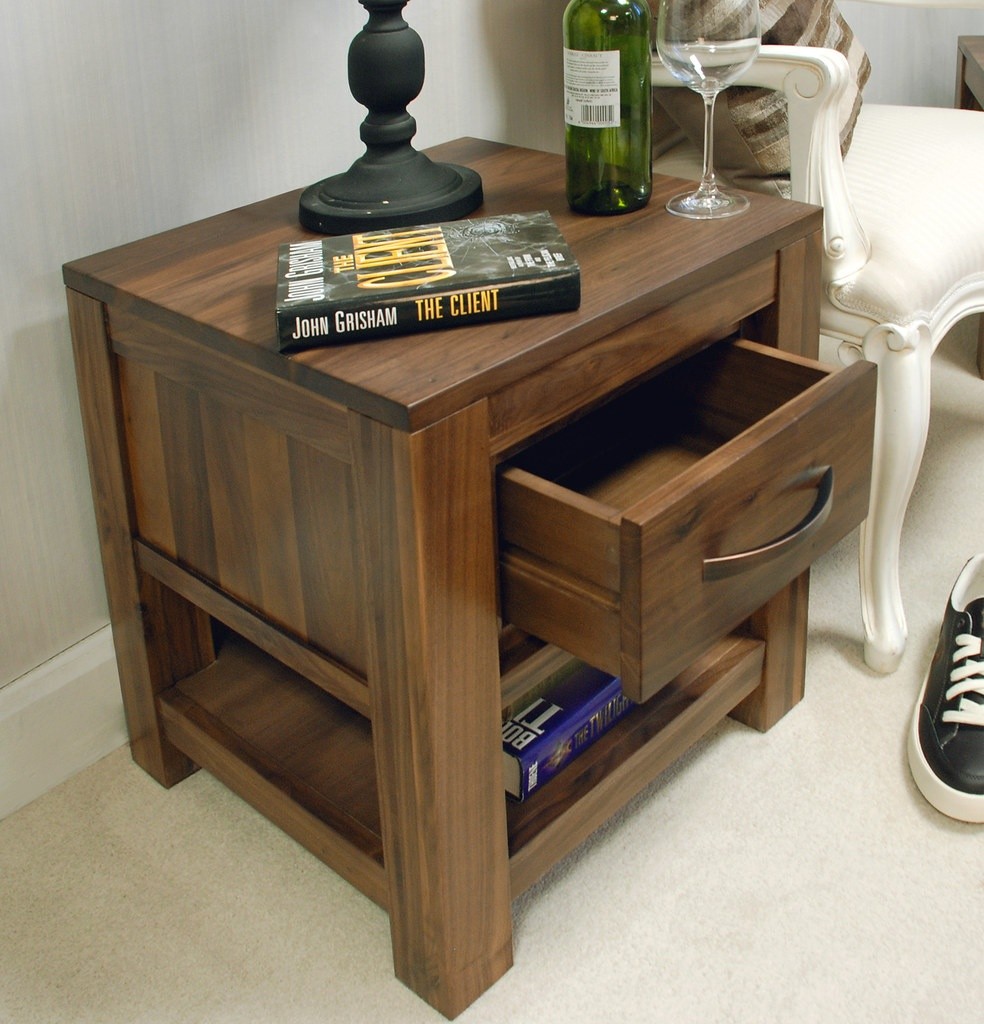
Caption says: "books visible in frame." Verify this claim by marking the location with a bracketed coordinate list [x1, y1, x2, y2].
[275, 208, 582, 353]
[502, 659, 636, 804]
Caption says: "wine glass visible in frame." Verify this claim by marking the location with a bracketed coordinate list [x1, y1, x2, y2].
[654, 0, 763, 218]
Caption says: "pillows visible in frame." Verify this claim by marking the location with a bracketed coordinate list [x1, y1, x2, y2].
[651, 0, 872, 204]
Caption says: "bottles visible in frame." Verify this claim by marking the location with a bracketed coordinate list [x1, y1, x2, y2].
[562, 0, 654, 214]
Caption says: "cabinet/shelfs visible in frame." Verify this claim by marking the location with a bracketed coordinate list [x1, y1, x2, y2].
[61, 136, 876, 1021]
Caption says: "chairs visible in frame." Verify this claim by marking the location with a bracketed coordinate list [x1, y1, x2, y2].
[648, 43, 984, 672]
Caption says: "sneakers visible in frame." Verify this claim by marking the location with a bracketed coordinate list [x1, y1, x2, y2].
[907, 552, 984, 824]
